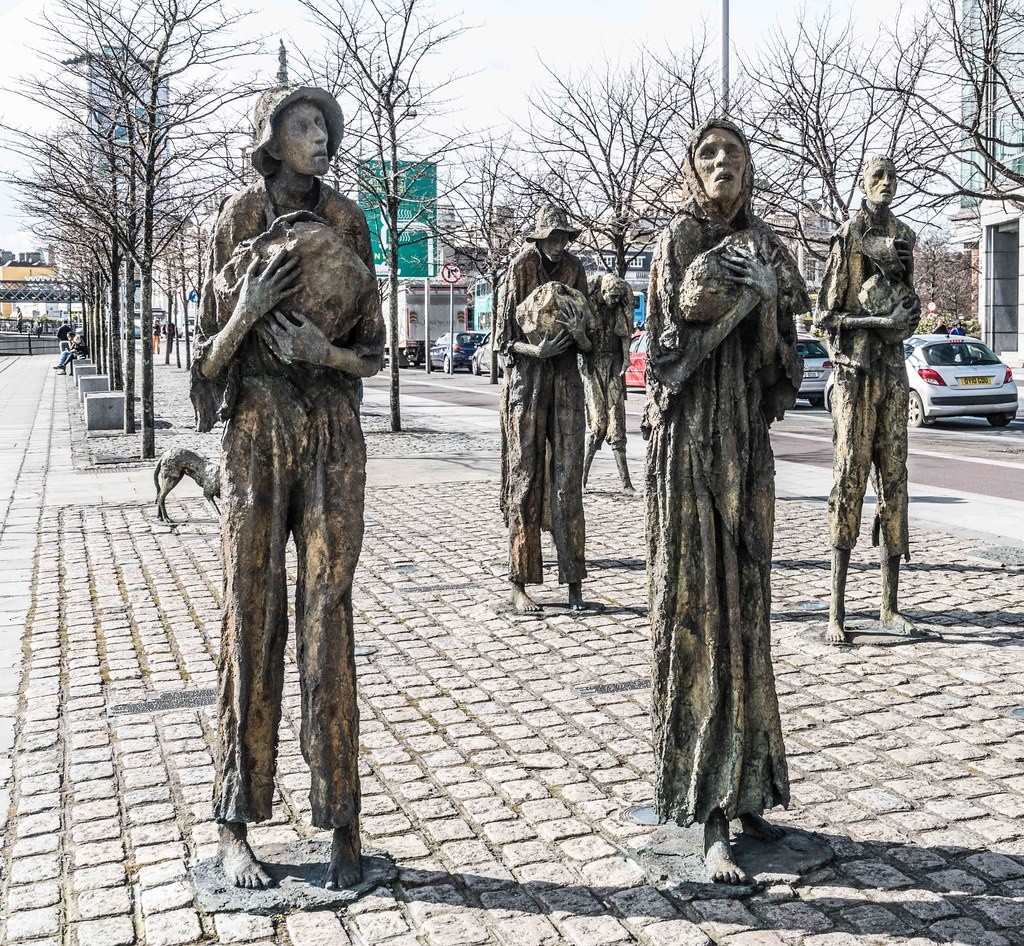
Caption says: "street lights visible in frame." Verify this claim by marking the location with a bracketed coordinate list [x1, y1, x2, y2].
[770, 115, 808, 340]
[377, 76, 417, 160]
[50, 281, 73, 327]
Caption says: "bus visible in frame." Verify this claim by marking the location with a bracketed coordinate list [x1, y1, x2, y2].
[631, 291, 645, 329]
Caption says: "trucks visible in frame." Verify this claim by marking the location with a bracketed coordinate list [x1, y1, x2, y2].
[379, 280, 469, 369]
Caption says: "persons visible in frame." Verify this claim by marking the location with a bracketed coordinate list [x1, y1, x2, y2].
[53, 320, 89, 375]
[812, 155, 920, 642]
[16, 308, 23, 334]
[934, 317, 966, 337]
[646, 119, 811, 883]
[152, 320, 176, 355]
[493, 205, 592, 611]
[583, 274, 637, 494]
[185, 89, 386, 890]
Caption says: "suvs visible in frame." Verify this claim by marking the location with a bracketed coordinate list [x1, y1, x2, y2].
[431, 332, 489, 374]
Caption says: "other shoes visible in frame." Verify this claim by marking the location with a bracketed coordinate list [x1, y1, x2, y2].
[58, 372, 66, 375]
[53, 366, 61, 369]
[58, 365, 65, 369]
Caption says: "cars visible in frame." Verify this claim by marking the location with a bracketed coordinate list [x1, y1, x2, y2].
[796, 330, 833, 408]
[624, 331, 648, 392]
[469, 330, 503, 378]
[823, 333, 1020, 429]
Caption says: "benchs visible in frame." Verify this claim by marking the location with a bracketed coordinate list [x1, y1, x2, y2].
[71, 358, 127, 431]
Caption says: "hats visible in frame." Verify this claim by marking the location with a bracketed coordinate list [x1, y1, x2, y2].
[75, 328, 83, 332]
